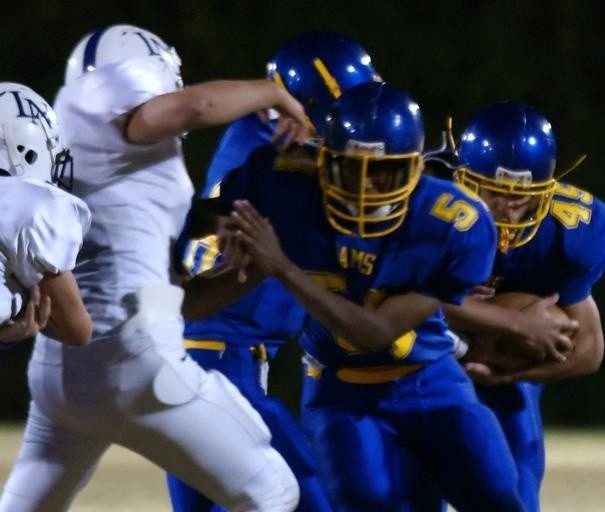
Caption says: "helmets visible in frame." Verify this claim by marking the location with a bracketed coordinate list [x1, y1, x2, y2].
[267, 33, 377, 134]
[65, 24, 185, 89]
[317, 82, 426, 239]
[454, 106, 558, 254]
[0, 82, 74, 194]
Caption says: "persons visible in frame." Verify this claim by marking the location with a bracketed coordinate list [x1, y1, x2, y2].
[0, 82, 93, 346]
[0, 24, 309, 512]
[167, 34, 385, 512]
[407, 102, 605, 512]
[218, 83, 526, 512]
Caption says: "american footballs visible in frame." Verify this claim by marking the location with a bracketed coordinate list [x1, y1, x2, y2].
[460, 290, 574, 370]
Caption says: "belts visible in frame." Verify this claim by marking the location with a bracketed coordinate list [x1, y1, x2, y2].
[306, 364, 425, 384]
[180, 339, 226, 351]
